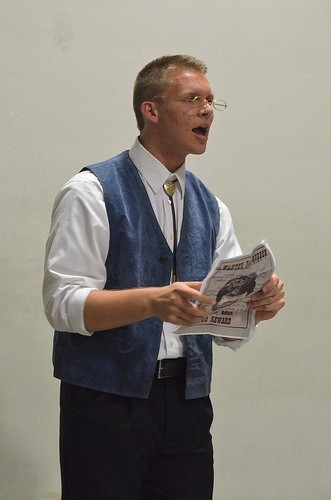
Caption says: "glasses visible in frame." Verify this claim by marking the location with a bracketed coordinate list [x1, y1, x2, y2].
[150, 94, 228, 111]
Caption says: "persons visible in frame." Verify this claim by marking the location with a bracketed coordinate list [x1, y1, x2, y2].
[41, 55, 288, 500]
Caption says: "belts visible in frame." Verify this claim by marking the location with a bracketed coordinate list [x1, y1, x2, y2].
[152, 357, 186, 378]
